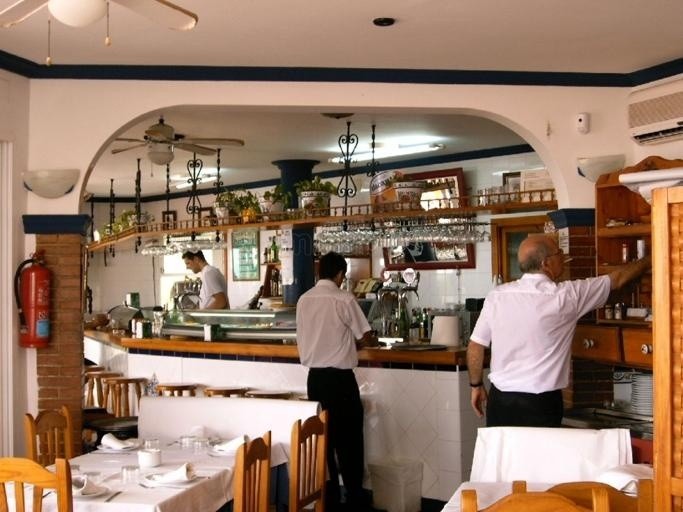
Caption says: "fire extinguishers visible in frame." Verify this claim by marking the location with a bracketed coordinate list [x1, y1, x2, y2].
[14, 248, 53, 348]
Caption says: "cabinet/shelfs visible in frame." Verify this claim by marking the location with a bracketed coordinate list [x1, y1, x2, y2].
[594, 154, 683, 326]
[569, 320, 653, 371]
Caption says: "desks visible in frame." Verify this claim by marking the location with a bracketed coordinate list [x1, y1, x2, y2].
[440, 480, 625, 511]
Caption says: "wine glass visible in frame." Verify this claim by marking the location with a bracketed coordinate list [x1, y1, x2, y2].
[316, 212, 501, 256]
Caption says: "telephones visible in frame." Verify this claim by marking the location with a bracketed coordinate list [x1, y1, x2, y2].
[354, 278, 376, 293]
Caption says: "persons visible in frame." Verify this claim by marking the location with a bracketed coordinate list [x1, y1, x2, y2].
[297, 251, 374, 511]
[464, 232, 652, 432]
[182, 249, 230, 312]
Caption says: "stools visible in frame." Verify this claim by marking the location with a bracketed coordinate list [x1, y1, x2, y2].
[82, 364, 311, 416]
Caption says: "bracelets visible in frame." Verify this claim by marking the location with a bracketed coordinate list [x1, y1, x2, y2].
[471, 381, 485, 389]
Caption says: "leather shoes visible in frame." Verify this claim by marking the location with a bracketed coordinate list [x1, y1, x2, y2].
[327, 481, 341, 497]
[344, 486, 372, 498]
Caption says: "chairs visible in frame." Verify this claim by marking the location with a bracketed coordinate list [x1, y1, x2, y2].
[0, 454, 72, 512]
[509, 477, 653, 512]
[231, 431, 271, 511]
[287, 410, 328, 511]
[24, 406, 72, 467]
[459, 486, 610, 512]
[471, 422, 633, 486]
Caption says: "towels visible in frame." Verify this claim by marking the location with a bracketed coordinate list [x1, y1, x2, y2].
[68, 476, 102, 496]
[215, 434, 250, 453]
[155, 460, 201, 483]
[99, 432, 133, 450]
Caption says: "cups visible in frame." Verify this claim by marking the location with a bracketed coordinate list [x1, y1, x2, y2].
[122, 435, 210, 491]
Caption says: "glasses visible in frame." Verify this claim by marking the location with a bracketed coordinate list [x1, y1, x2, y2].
[337, 271, 348, 283]
[539, 248, 564, 265]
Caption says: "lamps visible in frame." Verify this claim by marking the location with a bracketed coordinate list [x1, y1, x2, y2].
[577, 153, 625, 182]
[48, 1, 107, 29]
[325, 142, 446, 165]
[147, 143, 173, 166]
[22, 168, 81, 199]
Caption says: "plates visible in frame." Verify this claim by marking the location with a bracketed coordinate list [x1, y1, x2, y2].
[630, 372, 653, 416]
[65, 484, 110, 500]
[88, 437, 142, 453]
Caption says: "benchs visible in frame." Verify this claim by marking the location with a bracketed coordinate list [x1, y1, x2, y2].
[134, 395, 330, 440]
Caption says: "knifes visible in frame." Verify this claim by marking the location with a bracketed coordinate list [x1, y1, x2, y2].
[104, 489, 123, 504]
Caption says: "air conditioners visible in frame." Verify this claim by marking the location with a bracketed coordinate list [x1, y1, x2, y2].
[628, 77, 683, 147]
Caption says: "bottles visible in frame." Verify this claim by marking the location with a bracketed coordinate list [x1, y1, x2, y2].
[543, 220, 559, 233]
[394, 301, 434, 340]
[604, 297, 626, 319]
[264, 235, 282, 296]
[620, 240, 646, 264]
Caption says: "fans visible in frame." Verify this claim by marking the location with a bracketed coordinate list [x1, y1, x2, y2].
[0, 0, 198, 34]
[110, 113, 244, 157]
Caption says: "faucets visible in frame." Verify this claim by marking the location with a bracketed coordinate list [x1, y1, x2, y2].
[373, 270, 422, 337]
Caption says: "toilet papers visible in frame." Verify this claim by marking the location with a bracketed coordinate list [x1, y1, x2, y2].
[431, 315, 460, 349]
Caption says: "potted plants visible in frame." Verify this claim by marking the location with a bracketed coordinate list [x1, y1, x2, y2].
[95, 209, 155, 238]
[392, 176, 426, 210]
[213, 176, 339, 225]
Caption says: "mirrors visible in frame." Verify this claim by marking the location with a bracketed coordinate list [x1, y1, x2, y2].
[374, 166, 476, 271]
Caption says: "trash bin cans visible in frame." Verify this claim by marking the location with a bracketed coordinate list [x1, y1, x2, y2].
[367, 459, 423, 512]
